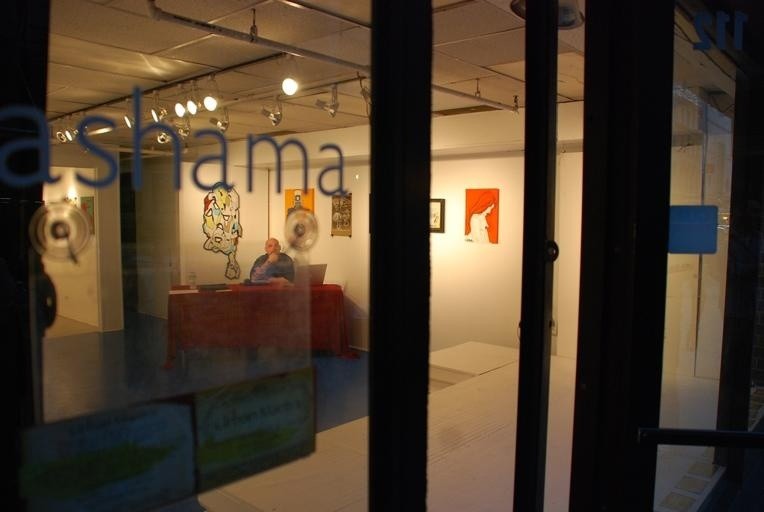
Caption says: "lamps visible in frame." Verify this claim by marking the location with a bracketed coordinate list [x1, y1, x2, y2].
[124, 111, 137, 129]
[316, 96, 339, 117]
[150, 107, 168, 123]
[203, 95, 224, 112]
[175, 102, 188, 118]
[174, 124, 191, 137]
[186, 98, 203, 115]
[57, 127, 68, 142]
[209, 113, 230, 133]
[259, 101, 283, 127]
[65, 127, 80, 142]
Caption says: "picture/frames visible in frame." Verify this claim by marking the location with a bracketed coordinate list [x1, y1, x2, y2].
[430, 199, 445, 233]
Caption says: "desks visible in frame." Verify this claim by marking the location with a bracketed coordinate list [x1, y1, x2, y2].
[165, 284, 359, 370]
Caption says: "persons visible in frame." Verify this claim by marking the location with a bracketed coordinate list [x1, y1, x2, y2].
[250, 239, 294, 282]
